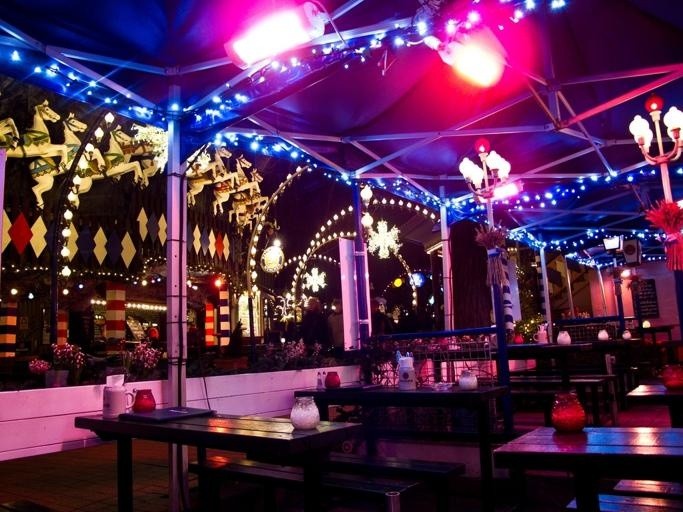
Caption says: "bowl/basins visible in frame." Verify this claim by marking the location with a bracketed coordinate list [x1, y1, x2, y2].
[426, 383, 453, 392]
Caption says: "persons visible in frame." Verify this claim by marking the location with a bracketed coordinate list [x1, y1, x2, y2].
[370, 299, 394, 335]
[299, 298, 345, 362]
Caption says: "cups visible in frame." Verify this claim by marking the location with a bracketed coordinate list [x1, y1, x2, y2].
[533, 331, 547, 343]
[103, 386, 135, 419]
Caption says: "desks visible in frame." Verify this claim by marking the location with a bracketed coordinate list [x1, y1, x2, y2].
[415, 343, 593, 427]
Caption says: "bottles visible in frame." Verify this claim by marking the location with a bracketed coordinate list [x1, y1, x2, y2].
[552, 393, 586, 432]
[316, 371, 326, 389]
[290, 396, 320, 430]
[324, 372, 340, 388]
[557, 331, 571, 344]
[459, 370, 478, 390]
[134, 389, 156, 412]
[398, 357, 416, 390]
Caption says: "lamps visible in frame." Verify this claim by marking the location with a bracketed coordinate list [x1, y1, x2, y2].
[603, 97, 682, 330]
[460, 143, 515, 440]
[224, 2, 326, 70]
